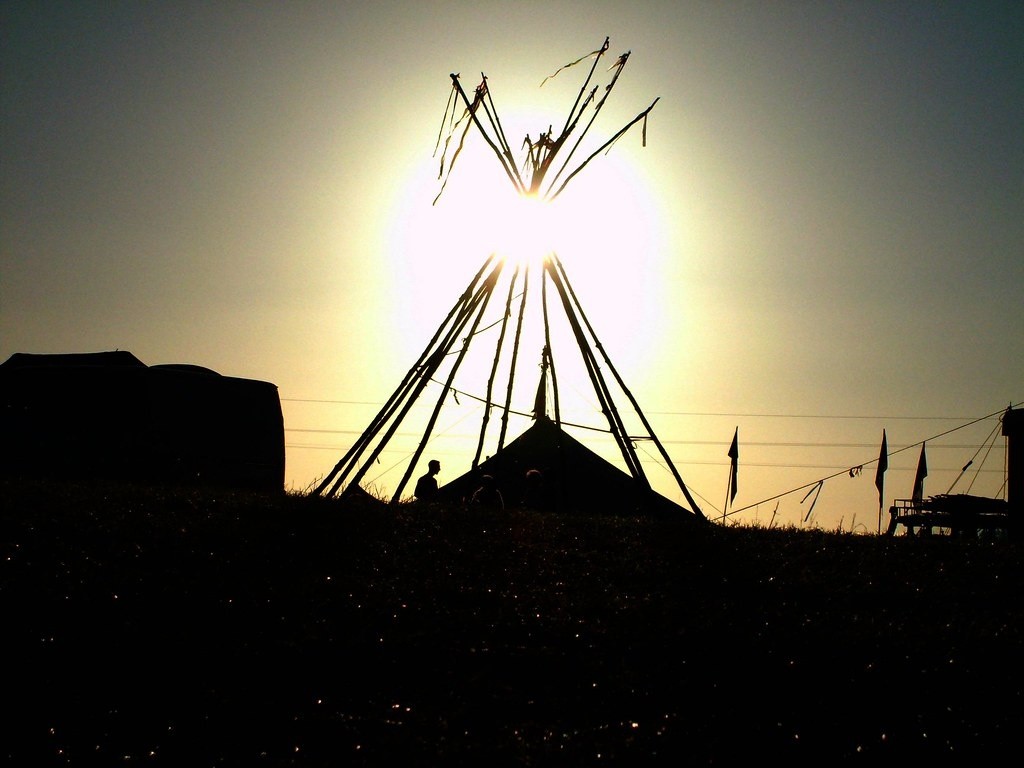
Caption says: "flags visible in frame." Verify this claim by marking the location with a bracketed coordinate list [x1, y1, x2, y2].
[875, 428, 887, 508]
[532, 369, 546, 421]
[912, 441, 927, 514]
[728, 426, 738, 505]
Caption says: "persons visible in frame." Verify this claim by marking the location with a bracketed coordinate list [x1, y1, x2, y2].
[470, 474, 504, 510]
[520, 470, 541, 513]
[414, 460, 440, 501]
